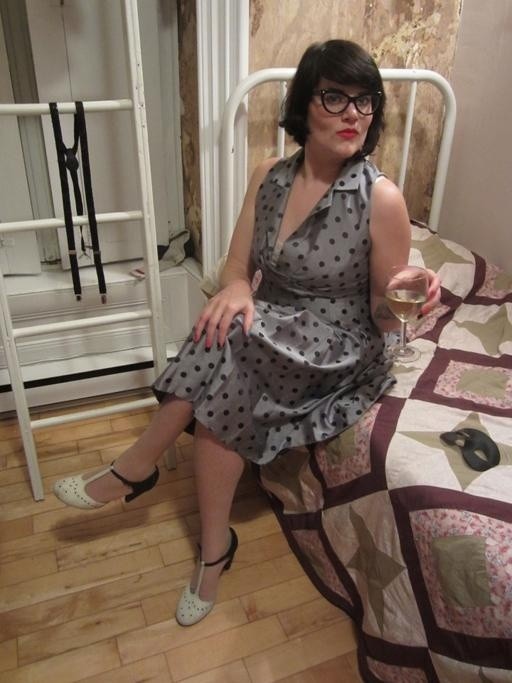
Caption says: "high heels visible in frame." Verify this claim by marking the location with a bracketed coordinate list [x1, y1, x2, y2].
[52, 460, 159, 509]
[175, 527, 238, 626]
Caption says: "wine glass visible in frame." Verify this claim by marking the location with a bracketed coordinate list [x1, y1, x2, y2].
[382, 264, 430, 365]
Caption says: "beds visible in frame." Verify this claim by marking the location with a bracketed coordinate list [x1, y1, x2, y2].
[195, 63, 512, 682]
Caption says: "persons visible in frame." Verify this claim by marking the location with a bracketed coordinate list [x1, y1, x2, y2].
[53, 40, 441, 627]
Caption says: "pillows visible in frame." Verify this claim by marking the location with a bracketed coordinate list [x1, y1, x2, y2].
[198, 216, 512, 341]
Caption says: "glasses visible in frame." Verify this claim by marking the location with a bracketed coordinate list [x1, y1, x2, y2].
[314, 89, 383, 115]
